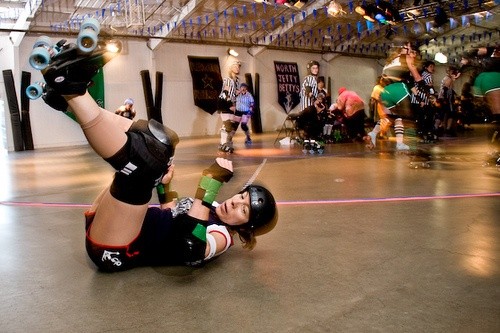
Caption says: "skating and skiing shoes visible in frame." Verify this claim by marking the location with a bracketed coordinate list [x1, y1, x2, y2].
[245, 138, 253, 144]
[302, 139, 326, 154]
[423, 132, 439, 144]
[408, 148, 432, 168]
[363, 131, 376, 151]
[322, 134, 352, 143]
[395, 142, 410, 150]
[217, 142, 234, 155]
[434, 125, 474, 136]
[27, 18, 123, 113]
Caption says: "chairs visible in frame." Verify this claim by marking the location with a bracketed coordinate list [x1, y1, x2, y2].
[273, 113, 303, 145]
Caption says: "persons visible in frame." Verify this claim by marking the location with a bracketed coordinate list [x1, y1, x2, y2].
[363, 38, 500, 170]
[25, 19, 278, 274]
[114, 98, 137, 120]
[300, 60, 367, 155]
[231, 83, 255, 144]
[216, 61, 242, 156]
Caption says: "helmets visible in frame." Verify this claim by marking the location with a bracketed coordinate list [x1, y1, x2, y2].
[124, 97, 134, 104]
[315, 101, 325, 111]
[247, 184, 278, 238]
[227, 59, 241, 65]
[306, 60, 320, 68]
[446, 66, 457, 74]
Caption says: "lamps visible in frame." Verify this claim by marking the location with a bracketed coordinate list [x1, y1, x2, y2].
[227, 48, 238, 57]
